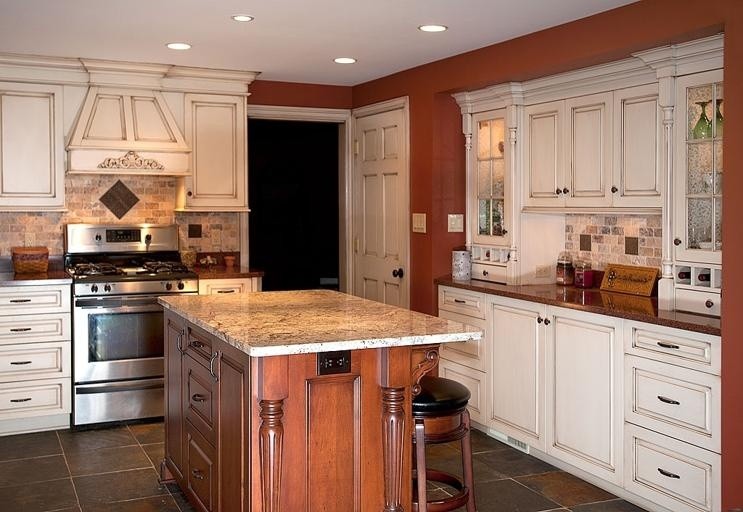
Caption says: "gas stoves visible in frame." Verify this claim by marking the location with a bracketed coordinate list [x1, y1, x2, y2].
[64, 223, 198, 297]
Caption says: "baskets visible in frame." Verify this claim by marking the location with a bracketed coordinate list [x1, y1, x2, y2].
[11, 247, 49, 273]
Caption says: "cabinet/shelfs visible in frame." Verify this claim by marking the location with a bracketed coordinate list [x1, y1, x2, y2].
[495, 293, 625, 506]
[611, 78, 662, 215]
[164, 302, 182, 490]
[181, 322, 213, 511]
[624, 313, 724, 512]
[175, 90, 249, 211]
[466, 102, 565, 284]
[434, 277, 487, 443]
[668, 75, 723, 305]
[0, 278, 72, 438]
[517, 91, 612, 213]
[213, 331, 250, 512]
[198, 273, 260, 297]
[0, 79, 65, 209]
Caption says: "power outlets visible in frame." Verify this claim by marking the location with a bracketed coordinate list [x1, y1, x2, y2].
[210, 224, 221, 245]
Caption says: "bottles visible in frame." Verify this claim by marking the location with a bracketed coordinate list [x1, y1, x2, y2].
[698, 274, 710, 281]
[678, 272, 690, 279]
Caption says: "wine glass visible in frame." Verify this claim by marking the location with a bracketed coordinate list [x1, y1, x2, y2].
[708, 99, 723, 137]
[693, 102, 711, 139]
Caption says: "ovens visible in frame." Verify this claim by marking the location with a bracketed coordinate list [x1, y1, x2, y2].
[72, 291, 198, 430]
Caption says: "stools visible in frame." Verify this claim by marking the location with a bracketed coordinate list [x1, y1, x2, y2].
[409, 374, 477, 512]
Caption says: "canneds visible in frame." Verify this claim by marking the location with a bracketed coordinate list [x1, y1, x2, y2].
[556, 260, 573, 285]
[574, 261, 592, 288]
